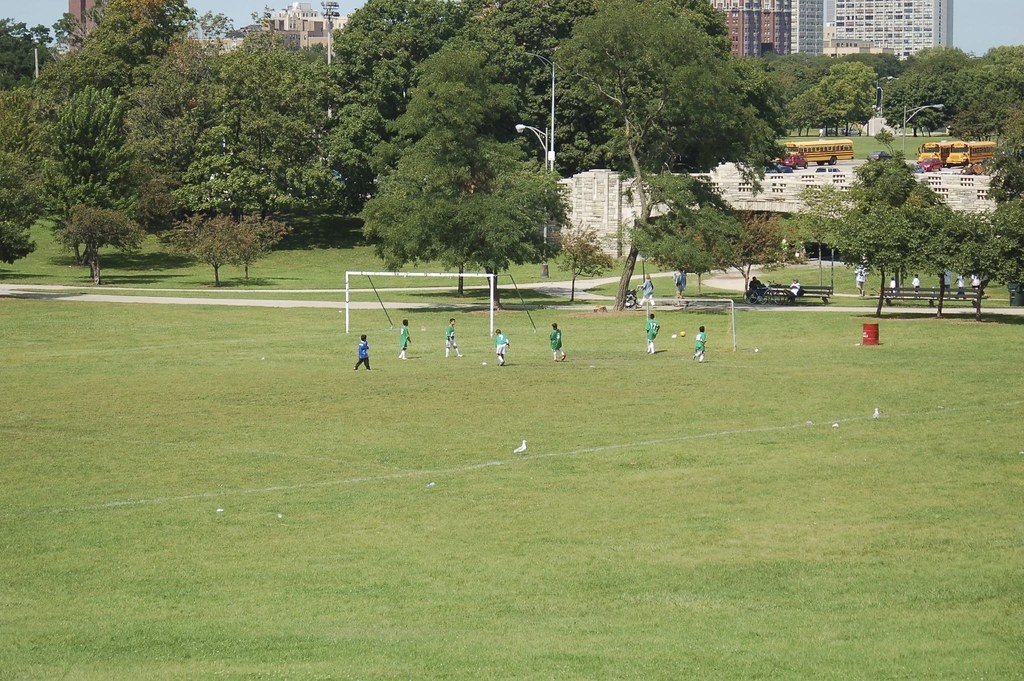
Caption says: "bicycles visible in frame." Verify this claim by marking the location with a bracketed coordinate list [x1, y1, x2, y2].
[742, 280, 787, 305]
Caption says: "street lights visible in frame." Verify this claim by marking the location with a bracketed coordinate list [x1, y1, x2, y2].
[901, 102, 945, 160]
[514, 123, 549, 277]
[320, 1, 342, 118]
[877, 87, 883, 128]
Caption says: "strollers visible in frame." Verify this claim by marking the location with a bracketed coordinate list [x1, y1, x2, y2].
[623, 286, 644, 309]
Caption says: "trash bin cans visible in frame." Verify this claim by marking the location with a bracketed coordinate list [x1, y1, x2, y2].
[1009, 287, 1024, 306]
[862, 323, 881, 346]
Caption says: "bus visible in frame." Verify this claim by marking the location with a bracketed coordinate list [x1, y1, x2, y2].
[945, 141, 997, 169]
[775, 139, 855, 166]
[917, 140, 964, 168]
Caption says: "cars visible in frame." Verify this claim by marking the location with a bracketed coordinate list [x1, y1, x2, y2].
[907, 163, 925, 174]
[763, 165, 794, 174]
[778, 153, 809, 170]
[815, 167, 842, 172]
[843, 127, 860, 135]
[866, 150, 893, 162]
[827, 128, 836, 135]
[918, 157, 946, 172]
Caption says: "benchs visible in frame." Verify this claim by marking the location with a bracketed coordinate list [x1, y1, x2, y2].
[749, 285, 833, 303]
[873, 287, 979, 308]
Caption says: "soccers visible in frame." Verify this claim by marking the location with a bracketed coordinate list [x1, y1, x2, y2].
[680, 331, 686, 337]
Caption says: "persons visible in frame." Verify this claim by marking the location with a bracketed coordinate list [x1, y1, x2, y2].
[749, 277, 761, 290]
[495, 329, 510, 366]
[912, 274, 920, 298]
[444, 318, 462, 358]
[820, 128, 823, 136]
[645, 314, 660, 354]
[784, 280, 800, 305]
[854, 266, 865, 297]
[944, 269, 951, 299]
[692, 326, 707, 363]
[638, 274, 655, 309]
[969, 275, 980, 288]
[549, 323, 566, 361]
[398, 320, 411, 359]
[673, 270, 686, 307]
[354, 335, 371, 370]
[890, 277, 896, 293]
[953, 272, 966, 299]
